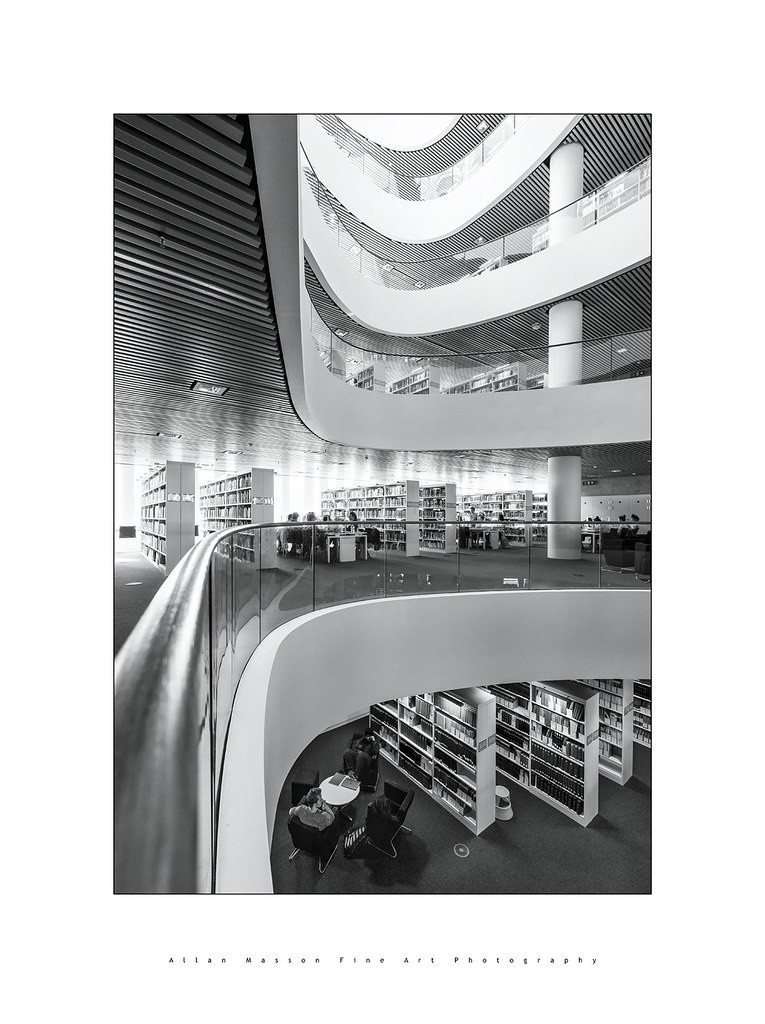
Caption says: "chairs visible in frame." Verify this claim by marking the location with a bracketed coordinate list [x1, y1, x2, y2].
[292, 767, 320, 806]
[287, 796, 344, 874]
[359, 528, 382, 559]
[339, 732, 380, 791]
[366, 779, 415, 858]
[600, 539, 651, 584]
[276, 526, 325, 564]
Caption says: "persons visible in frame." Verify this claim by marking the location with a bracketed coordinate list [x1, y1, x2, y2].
[498, 514, 507, 527]
[289, 728, 390, 831]
[586, 514, 639, 547]
[470, 507, 478, 527]
[284, 512, 358, 554]
[479, 513, 486, 527]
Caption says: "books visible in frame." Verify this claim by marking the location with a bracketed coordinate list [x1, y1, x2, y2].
[328, 773, 346, 786]
[320, 348, 544, 397]
[322, 486, 446, 550]
[138, 469, 255, 568]
[457, 492, 547, 542]
[379, 677, 652, 816]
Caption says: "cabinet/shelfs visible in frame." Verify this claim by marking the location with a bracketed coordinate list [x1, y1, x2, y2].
[443, 362, 528, 395]
[632, 679, 652, 748]
[139, 460, 195, 577]
[369, 688, 495, 836]
[345, 361, 390, 393]
[387, 365, 440, 395]
[419, 484, 457, 554]
[321, 480, 419, 557]
[527, 373, 547, 390]
[200, 468, 278, 570]
[456, 489, 533, 547]
[479, 681, 600, 828]
[572, 679, 633, 786]
[532, 493, 548, 548]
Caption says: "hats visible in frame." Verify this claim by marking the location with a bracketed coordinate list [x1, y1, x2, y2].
[364, 728, 374, 737]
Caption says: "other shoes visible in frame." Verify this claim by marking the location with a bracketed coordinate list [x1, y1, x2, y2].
[289, 550, 300, 556]
[346, 771, 358, 781]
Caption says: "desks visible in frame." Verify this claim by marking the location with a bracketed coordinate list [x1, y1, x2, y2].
[581, 532, 600, 554]
[324, 532, 368, 562]
[319, 776, 360, 806]
[469, 527, 486, 551]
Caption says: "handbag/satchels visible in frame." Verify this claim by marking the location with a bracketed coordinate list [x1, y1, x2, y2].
[375, 794, 391, 816]
